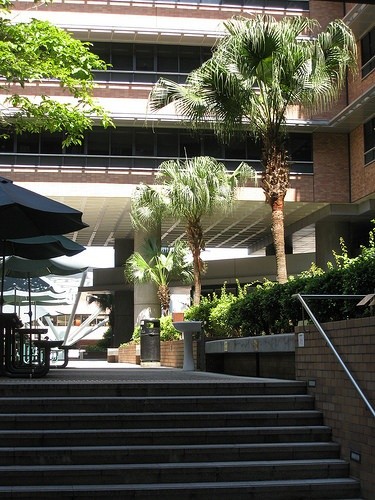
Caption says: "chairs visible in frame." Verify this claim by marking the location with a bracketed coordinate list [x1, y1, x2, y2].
[0, 311, 73, 378]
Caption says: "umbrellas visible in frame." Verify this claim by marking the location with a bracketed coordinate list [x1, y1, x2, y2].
[0, 175, 90, 377]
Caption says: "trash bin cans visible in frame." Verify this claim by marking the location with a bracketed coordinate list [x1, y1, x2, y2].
[139, 318, 162, 367]
